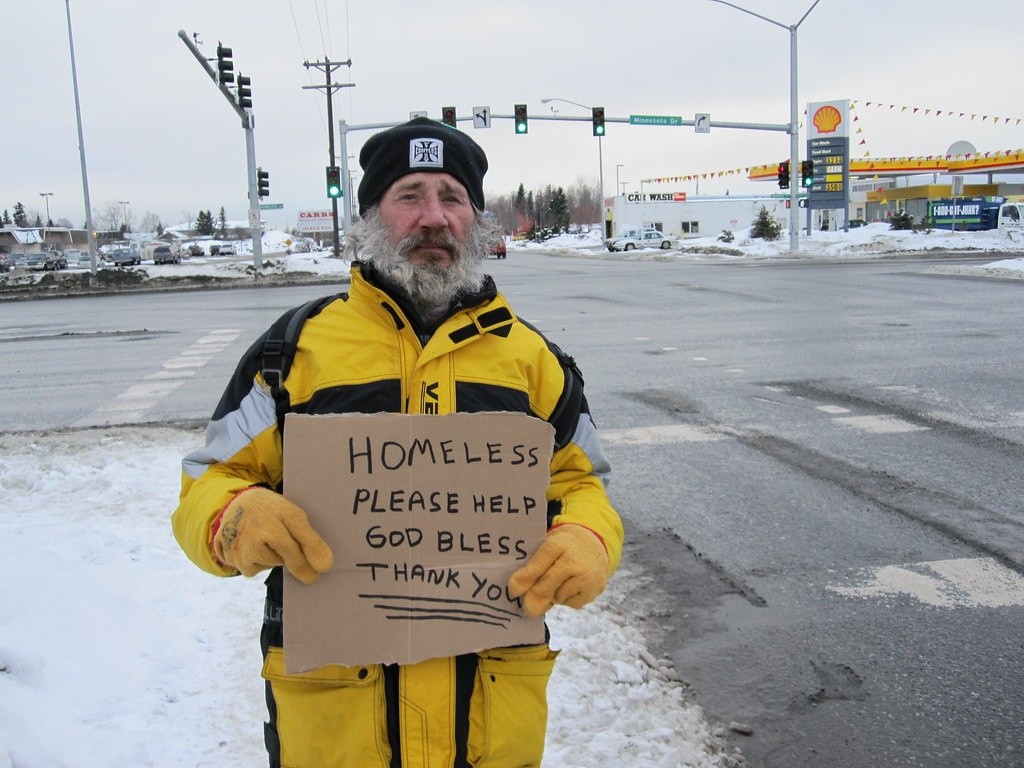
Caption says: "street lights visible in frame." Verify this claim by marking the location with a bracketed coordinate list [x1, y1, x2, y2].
[119, 202, 129, 246]
[40, 193, 54, 252]
[617, 165, 648, 250]
[541, 98, 606, 249]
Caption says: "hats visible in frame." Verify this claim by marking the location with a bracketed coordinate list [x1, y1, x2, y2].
[357, 117, 488, 219]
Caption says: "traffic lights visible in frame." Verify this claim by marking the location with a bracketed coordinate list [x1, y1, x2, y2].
[778, 163, 789, 189]
[257, 171, 269, 197]
[802, 161, 813, 188]
[514, 104, 528, 134]
[592, 107, 605, 135]
[326, 167, 341, 198]
[217, 41, 234, 83]
[442, 107, 456, 128]
[237, 71, 253, 108]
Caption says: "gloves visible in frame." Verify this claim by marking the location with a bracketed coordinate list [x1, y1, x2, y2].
[507, 523, 609, 618]
[213, 487, 334, 584]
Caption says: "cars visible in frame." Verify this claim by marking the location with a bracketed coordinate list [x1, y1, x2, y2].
[189, 244, 237, 256]
[485, 234, 506, 258]
[153, 247, 181, 265]
[604, 228, 679, 252]
[78, 249, 141, 269]
[0, 253, 68, 273]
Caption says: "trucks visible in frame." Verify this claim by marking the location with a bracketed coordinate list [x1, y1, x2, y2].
[927, 196, 1024, 232]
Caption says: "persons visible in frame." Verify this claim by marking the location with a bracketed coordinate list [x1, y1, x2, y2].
[172, 116, 625, 768]
[1006, 208, 1018, 222]
[821, 217, 830, 231]
